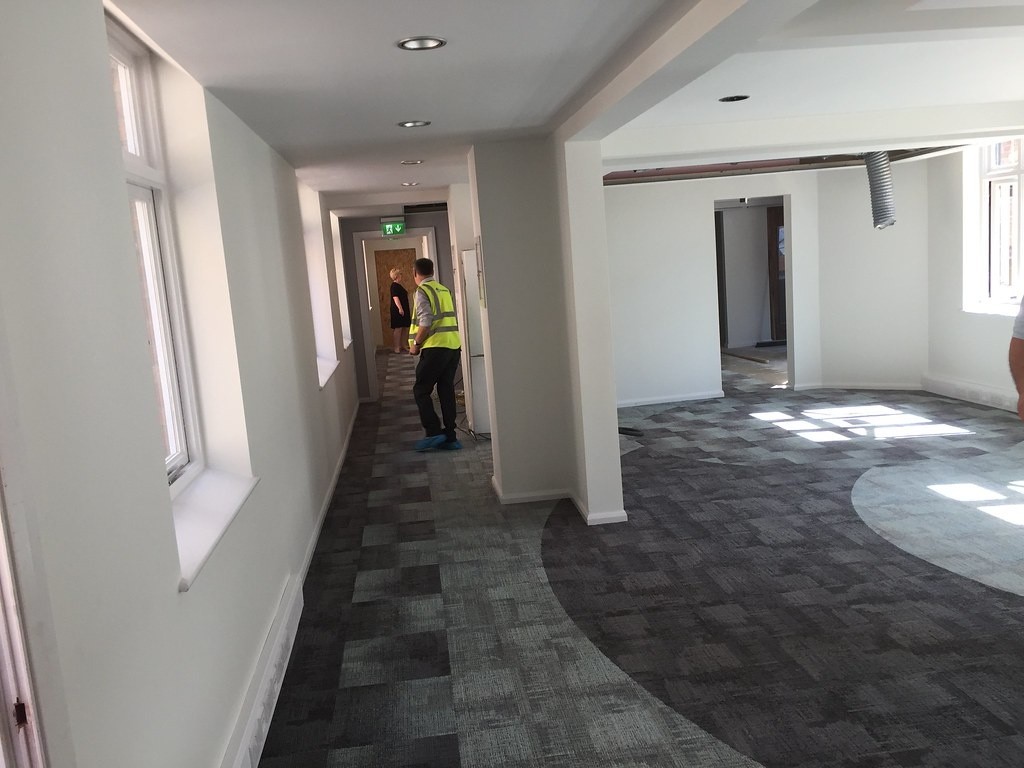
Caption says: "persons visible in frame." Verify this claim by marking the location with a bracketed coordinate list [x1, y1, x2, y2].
[390, 268, 409, 353]
[408, 258, 461, 451]
[1009, 295, 1024, 419]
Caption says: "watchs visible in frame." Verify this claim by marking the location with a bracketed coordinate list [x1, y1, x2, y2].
[413, 340, 421, 346]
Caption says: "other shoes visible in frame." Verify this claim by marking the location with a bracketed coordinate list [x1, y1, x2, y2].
[395, 348, 399, 354]
[444, 440, 462, 450]
[415, 434, 446, 450]
[401, 347, 411, 351]
